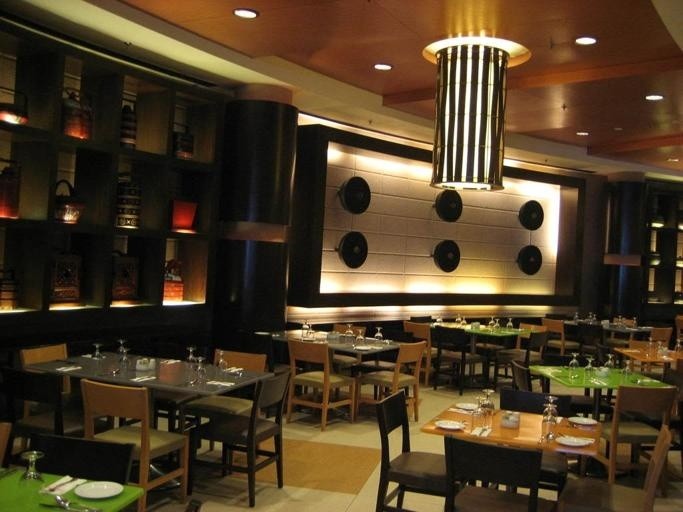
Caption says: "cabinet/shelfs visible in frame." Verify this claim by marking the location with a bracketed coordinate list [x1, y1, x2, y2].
[0, 15, 227, 339]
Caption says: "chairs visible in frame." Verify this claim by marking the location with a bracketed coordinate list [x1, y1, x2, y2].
[3, 343, 112, 470]
[0, 422, 12, 468]
[181, 350, 266, 476]
[30, 432, 136, 486]
[193, 369, 292, 507]
[79, 378, 189, 509]
[151, 333, 210, 364]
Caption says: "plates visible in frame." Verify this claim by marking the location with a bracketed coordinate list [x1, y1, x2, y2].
[75, 481, 124, 499]
[569, 417, 598, 426]
[556, 436, 595, 446]
[435, 420, 464, 430]
[456, 403, 478, 410]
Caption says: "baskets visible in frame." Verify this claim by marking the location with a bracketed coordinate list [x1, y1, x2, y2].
[53, 180, 83, 224]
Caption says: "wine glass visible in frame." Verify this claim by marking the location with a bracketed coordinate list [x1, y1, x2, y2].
[19, 451, 44, 495]
[185, 347, 227, 380]
[471, 389, 558, 439]
[573, 312, 637, 328]
[92, 338, 129, 368]
[569, 353, 632, 378]
[435, 312, 513, 334]
[646, 338, 683, 359]
[302, 319, 383, 344]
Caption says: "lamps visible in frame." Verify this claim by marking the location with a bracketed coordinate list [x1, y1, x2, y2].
[422, 36, 532, 190]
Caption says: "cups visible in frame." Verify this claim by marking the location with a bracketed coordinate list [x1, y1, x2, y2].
[136, 358, 155, 370]
